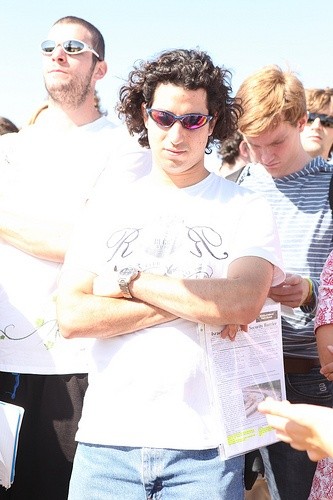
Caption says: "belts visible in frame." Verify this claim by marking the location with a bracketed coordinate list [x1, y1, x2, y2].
[284, 358, 321, 374]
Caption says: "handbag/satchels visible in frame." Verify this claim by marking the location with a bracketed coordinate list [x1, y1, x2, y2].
[0, 401, 25, 489]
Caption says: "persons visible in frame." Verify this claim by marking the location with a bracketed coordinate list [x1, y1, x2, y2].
[0, 16, 153, 500]
[55, 49, 285, 500]
[215, 67, 333, 500]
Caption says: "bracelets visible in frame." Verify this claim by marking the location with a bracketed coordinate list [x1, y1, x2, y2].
[303, 277, 313, 304]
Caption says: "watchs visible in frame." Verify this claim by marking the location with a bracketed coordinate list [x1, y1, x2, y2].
[118, 267, 140, 299]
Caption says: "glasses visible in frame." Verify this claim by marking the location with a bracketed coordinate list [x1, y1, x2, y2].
[39, 40, 102, 61]
[146, 108, 214, 130]
[308, 113, 333, 127]
